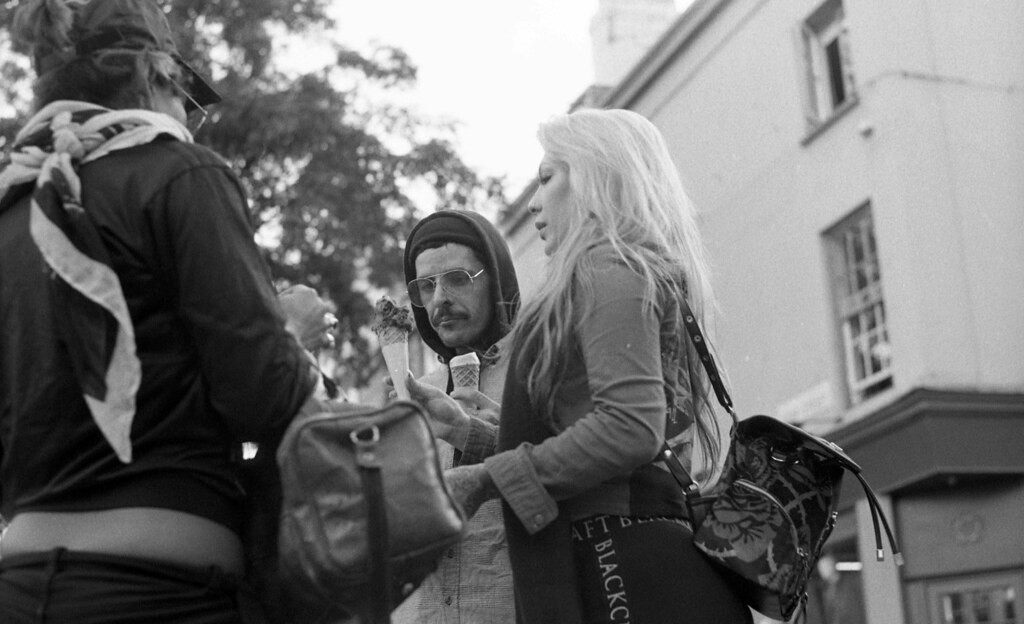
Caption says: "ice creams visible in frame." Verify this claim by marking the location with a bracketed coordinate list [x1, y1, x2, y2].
[372, 297, 414, 401]
[450, 352, 481, 411]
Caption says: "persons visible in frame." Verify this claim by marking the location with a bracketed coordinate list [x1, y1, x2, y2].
[383, 108, 754, 624]
[0, 0, 341, 624]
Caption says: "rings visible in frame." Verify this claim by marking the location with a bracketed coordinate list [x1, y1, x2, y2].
[325, 332, 334, 349]
[325, 312, 339, 329]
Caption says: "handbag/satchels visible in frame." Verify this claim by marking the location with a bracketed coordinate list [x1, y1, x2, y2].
[664, 265, 903, 623]
[274, 358, 467, 601]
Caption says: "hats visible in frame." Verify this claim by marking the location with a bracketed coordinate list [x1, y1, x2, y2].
[34, 0, 222, 115]
[410, 218, 481, 265]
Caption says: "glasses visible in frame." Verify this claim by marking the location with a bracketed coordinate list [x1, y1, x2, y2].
[407, 268, 486, 307]
[170, 77, 208, 136]
